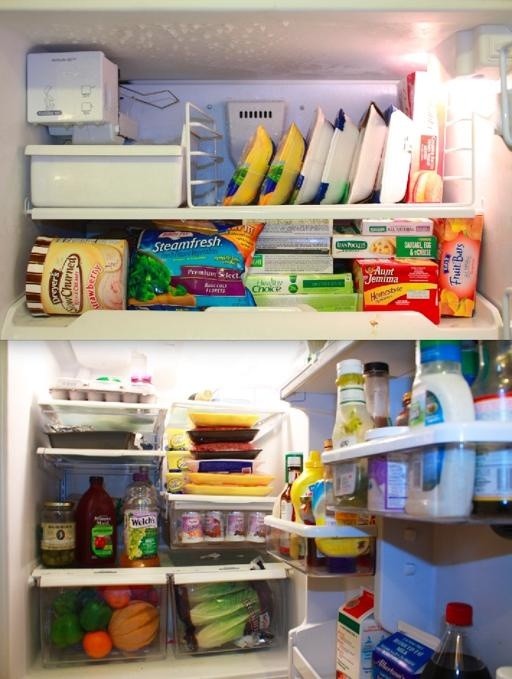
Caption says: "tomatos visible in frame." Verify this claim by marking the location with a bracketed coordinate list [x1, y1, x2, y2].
[97, 585, 158, 609]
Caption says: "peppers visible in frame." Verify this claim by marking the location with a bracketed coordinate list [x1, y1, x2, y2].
[50, 588, 112, 650]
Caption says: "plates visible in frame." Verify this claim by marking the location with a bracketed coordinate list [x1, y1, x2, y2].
[181, 411, 275, 496]
[221, 105, 412, 204]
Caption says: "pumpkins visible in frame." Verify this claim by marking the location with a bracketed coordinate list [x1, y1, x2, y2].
[108, 599, 159, 652]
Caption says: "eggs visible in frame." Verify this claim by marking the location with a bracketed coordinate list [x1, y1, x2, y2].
[51, 387, 156, 404]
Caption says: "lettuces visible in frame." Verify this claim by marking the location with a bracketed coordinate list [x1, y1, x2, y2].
[187, 581, 263, 649]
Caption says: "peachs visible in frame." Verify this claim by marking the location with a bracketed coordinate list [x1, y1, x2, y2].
[412, 171, 443, 203]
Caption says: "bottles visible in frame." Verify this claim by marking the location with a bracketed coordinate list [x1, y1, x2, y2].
[421, 602, 493, 678]
[326, 354, 378, 507]
[362, 359, 398, 430]
[467, 339, 511, 516]
[118, 462, 162, 567]
[277, 469, 301, 551]
[299, 494, 326, 567]
[77, 474, 118, 567]
[315, 438, 372, 559]
[289, 450, 324, 560]
[402, 339, 477, 520]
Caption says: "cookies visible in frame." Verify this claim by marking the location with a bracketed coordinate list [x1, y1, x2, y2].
[370, 239, 394, 255]
